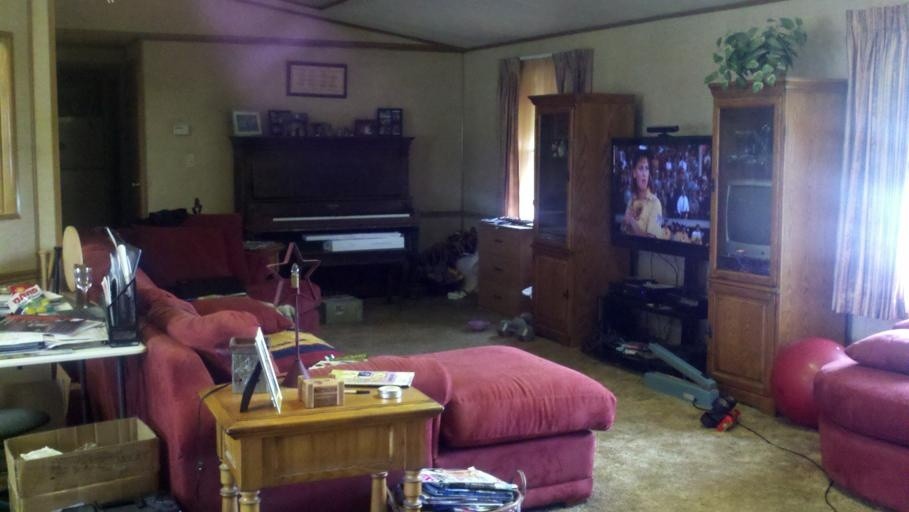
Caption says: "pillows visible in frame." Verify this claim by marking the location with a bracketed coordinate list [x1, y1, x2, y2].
[842, 327, 908, 375]
[128, 264, 340, 385]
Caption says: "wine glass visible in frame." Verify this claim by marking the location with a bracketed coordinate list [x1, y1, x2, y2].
[73, 264, 95, 311]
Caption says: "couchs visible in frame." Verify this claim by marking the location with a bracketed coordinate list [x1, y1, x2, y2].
[813, 315, 909, 511]
[65, 209, 454, 512]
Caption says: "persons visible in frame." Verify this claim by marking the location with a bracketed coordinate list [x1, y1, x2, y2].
[612, 141, 710, 246]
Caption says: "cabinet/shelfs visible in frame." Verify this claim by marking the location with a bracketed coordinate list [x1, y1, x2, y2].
[707, 75, 850, 421]
[476, 214, 532, 324]
[526, 91, 635, 350]
[582, 279, 707, 381]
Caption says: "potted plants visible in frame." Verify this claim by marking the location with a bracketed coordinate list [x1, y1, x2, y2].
[702, 15, 809, 95]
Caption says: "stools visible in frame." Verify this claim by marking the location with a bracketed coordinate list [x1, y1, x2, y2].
[402, 344, 618, 512]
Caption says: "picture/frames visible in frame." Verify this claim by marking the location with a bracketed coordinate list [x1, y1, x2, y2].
[239, 326, 284, 417]
[284, 57, 349, 101]
[230, 105, 263, 137]
[268, 105, 406, 139]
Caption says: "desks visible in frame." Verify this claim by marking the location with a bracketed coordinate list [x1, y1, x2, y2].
[0, 289, 149, 425]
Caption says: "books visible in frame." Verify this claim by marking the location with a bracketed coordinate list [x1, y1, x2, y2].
[325, 368, 416, 389]
[0, 314, 110, 351]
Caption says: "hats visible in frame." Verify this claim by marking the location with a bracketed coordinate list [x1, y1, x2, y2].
[688, 181, 698, 190]
[677, 196, 690, 216]
[678, 159, 687, 172]
[665, 162, 672, 170]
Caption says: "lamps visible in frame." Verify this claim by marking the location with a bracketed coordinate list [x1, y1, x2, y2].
[266, 241, 323, 389]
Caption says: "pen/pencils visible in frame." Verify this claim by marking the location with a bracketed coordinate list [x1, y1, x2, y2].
[342, 389, 371, 395]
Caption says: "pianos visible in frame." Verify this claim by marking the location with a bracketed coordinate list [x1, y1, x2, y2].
[229, 136, 420, 303]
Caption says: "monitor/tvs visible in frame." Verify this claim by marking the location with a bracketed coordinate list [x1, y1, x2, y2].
[722, 179, 773, 260]
[610, 136, 712, 261]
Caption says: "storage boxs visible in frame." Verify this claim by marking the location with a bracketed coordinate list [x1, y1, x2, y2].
[2, 415, 160, 512]
[0, 362, 72, 493]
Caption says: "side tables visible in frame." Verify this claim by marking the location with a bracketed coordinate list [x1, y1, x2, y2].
[198, 377, 447, 512]
